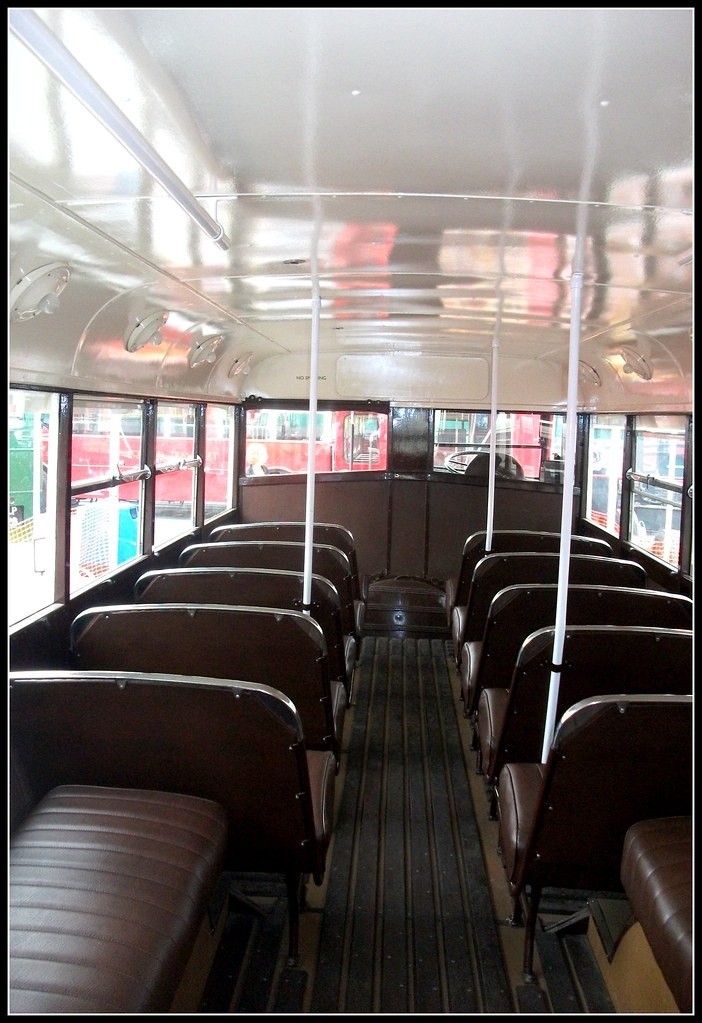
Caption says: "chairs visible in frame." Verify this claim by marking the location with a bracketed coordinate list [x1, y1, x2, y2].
[466, 453, 525, 479]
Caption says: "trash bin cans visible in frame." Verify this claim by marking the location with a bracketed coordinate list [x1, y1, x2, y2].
[81, 502, 139, 569]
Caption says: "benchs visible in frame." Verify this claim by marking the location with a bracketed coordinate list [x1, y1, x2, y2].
[446, 530, 614, 629]
[10, 670, 337, 966]
[476, 624, 692, 820]
[460, 583, 692, 720]
[132, 566, 358, 705]
[68, 603, 347, 766]
[11, 736, 228, 1013]
[495, 694, 692, 983]
[208, 523, 369, 604]
[178, 540, 366, 662]
[619, 816, 692, 1013]
[451, 552, 647, 674]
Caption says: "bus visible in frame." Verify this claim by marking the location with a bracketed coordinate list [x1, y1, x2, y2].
[8, 393, 554, 523]
